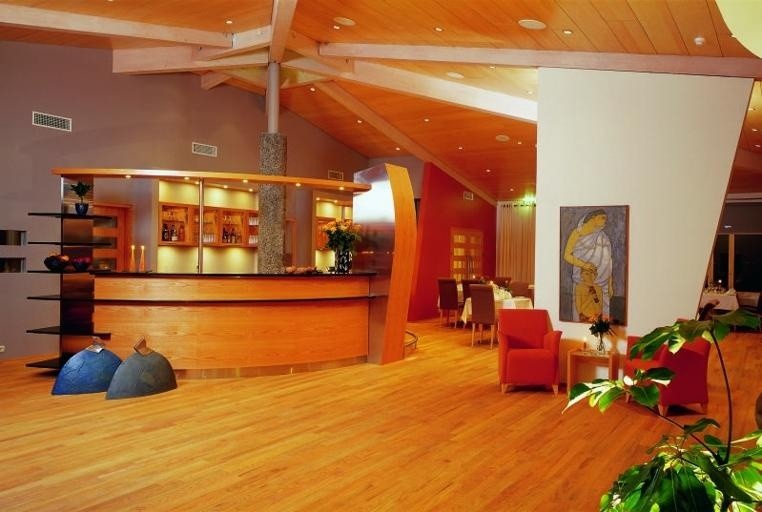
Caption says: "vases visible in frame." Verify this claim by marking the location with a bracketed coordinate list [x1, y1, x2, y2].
[596, 336, 607, 356]
[334, 248, 350, 274]
[73, 262, 91, 272]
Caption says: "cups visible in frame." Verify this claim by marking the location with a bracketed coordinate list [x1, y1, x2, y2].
[248, 235, 257, 243]
[196, 232, 216, 243]
[163, 209, 177, 220]
[248, 215, 259, 226]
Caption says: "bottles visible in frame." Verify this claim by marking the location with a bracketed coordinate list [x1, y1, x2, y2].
[224, 228, 242, 243]
[128, 245, 137, 273]
[138, 246, 145, 273]
[162, 221, 185, 241]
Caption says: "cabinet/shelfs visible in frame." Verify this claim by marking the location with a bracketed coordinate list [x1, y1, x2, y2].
[21, 211, 119, 372]
[246, 210, 259, 248]
[194, 205, 222, 246]
[222, 207, 247, 247]
[160, 202, 191, 246]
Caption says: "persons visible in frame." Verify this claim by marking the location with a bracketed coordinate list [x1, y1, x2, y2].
[572, 261, 604, 324]
[564, 209, 615, 330]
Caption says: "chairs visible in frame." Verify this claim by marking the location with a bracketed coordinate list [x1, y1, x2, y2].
[485, 274, 531, 298]
[496, 305, 563, 396]
[734, 291, 762, 334]
[462, 279, 480, 306]
[438, 275, 463, 330]
[468, 284, 498, 350]
[622, 329, 713, 418]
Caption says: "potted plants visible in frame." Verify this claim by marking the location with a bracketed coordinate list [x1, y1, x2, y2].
[70, 181, 95, 215]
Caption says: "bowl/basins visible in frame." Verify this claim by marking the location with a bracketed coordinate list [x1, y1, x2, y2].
[72, 261, 91, 271]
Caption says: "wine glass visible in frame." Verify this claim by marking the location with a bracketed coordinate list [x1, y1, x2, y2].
[223, 216, 240, 224]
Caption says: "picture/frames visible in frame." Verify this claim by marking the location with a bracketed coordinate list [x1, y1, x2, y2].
[558, 203, 630, 329]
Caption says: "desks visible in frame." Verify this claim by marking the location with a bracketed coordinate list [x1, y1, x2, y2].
[564, 344, 620, 403]
[454, 284, 499, 302]
[472, 295, 534, 345]
[697, 284, 740, 328]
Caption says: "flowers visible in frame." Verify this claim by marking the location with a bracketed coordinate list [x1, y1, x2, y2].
[69, 257, 93, 263]
[320, 218, 363, 251]
[588, 311, 619, 340]
